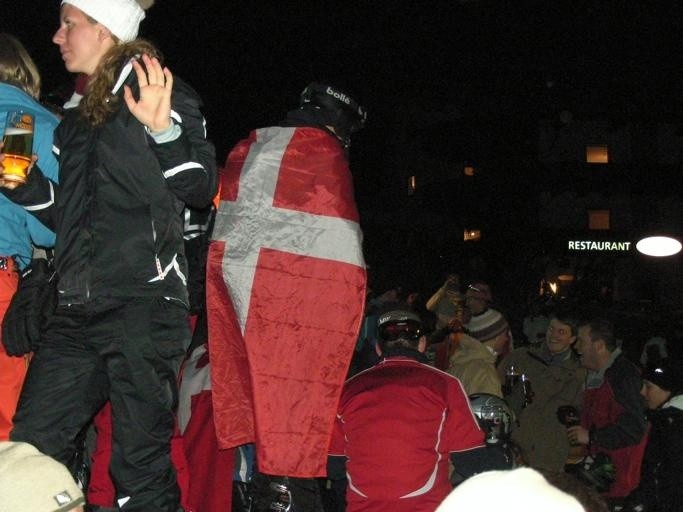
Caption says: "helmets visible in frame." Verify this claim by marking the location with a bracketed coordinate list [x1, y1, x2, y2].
[287, 81, 376, 150]
[468, 393, 520, 446]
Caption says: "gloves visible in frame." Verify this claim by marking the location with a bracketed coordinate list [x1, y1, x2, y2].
[0, 257, 58, 357]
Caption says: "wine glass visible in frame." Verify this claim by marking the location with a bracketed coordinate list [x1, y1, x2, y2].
[521, 373, 534, 407]
[503, 362, 518, 396]
[566, 412, 577, 449]
[481, 406, 503, 444]
[0, 111, 34, 183]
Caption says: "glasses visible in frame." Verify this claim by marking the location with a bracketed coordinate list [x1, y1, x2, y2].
[380, 320, 425, 342]
[469, 284, 481, 293]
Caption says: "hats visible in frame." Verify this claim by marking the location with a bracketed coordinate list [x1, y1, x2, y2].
[0, 442, 86, 512]
[62, 0, 154, 42]
[469, 307, 508, 341]
[377, 311, 423, 325]
[641, 357, 678, 391]
[464, 284, 493, 300]
